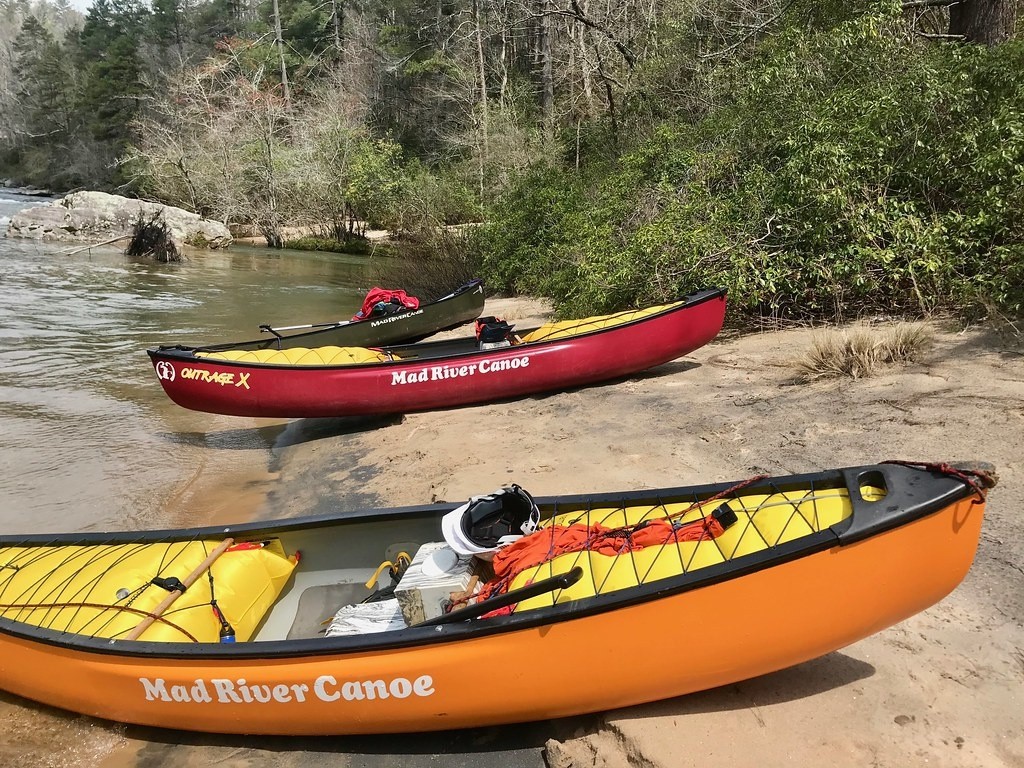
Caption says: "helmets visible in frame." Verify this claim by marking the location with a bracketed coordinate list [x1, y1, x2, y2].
[441, 485, 541, 563]
[474, 315, 516, 342]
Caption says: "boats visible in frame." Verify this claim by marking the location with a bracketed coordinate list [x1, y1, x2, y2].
[196, 279, 484, 352]
[1, 458, 1000, 736]
[147, 287, 728, 417]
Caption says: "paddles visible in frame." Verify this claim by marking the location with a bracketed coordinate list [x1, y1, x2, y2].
[400, 564, 585, 631]
[258, 316, 362, 339]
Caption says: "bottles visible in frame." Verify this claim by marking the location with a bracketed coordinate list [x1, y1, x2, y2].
[220, 622, 236, 643]
